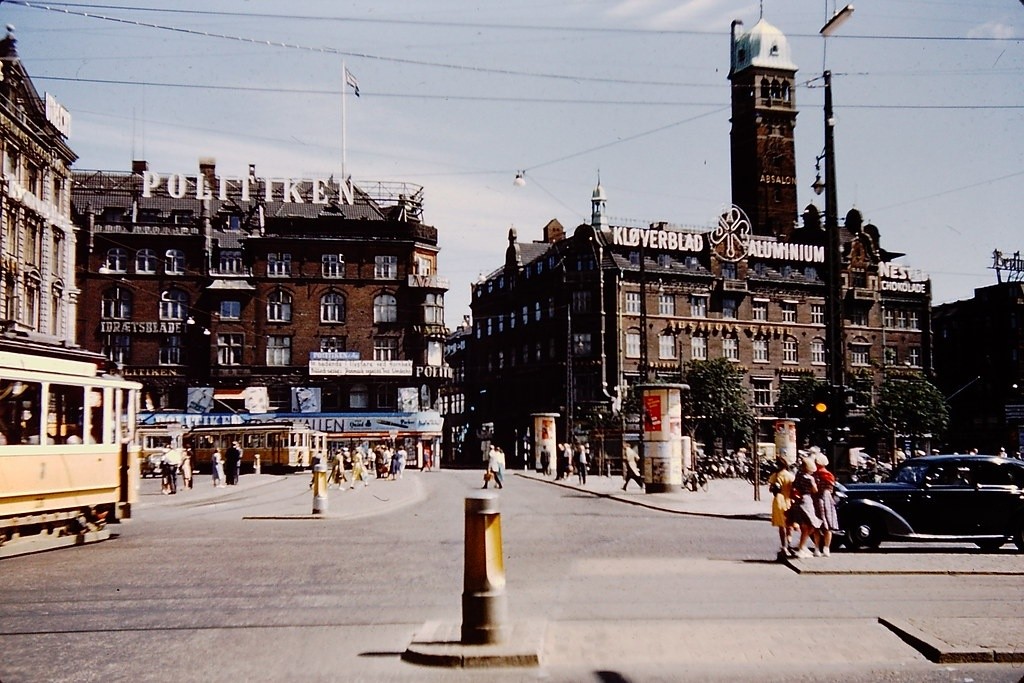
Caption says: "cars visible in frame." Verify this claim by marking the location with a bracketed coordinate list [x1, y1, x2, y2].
[809, 453, 1024, 557]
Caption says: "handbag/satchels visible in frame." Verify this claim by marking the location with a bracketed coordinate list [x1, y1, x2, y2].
[485, 474, 493, 480]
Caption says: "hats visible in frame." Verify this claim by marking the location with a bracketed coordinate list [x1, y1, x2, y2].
[816, 453, 829, 466]
[802, 458, 817, 472]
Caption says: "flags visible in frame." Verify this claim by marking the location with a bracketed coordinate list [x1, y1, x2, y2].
[345, 68, 360, 96]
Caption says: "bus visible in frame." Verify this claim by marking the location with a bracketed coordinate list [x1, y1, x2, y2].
[1, 324, 141, 561]
[311, 430, 328, 472]
[181, 420, 311, 473]
[136, 427, 176, 474]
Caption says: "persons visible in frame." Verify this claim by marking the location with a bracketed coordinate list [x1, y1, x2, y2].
[158, 436, 243, 495]
[308, 440, 434, 490]
[0, 391, 134, 445]
[886, 447, 1024, 465]
[481, 445, 506, 489]
[696, 445, 839, 559]
[540, 442, 646, 491]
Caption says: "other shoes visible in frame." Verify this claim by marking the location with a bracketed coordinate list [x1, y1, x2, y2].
[162, 489, 176, 494]
[639, 484, 645, 488]
[822, 546, 830, 557]
[327, 485, 347, 490]
[310, 484, 312, 487]
[496, 486, 502, 489]
[481, 487, 487, 489]
[782, 547, 798, 558]
[812, 549, 823, 557]
[377, 475, 402, 481]
[795, 548, 813, 557]
[349, 482, 370, 489]
[621, 488, 627, 492]
